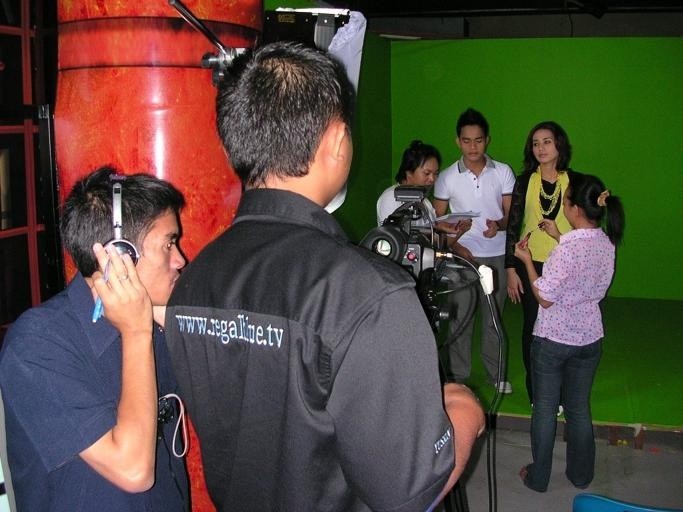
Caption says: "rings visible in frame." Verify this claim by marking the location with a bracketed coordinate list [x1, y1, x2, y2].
[119, 273, 130, 284]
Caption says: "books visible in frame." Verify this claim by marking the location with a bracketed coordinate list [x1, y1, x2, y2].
[430, 210, 479, 238]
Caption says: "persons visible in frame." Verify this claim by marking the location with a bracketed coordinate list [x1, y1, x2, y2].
[504, 121, 582, 416]
[374, 139, 472, 251]
[162, 39, 487, 511]
[511, 172, 625, 494]
[0, 162, 188, 512]
[431, 107, 517, 397]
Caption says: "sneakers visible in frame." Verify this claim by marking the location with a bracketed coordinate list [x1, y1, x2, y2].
[520, 467, 547, 492]
[495, 381, 512, 393]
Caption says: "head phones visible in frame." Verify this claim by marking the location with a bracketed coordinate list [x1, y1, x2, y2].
[97, 169, 140, 269]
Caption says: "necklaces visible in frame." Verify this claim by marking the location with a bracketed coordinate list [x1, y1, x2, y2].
[538, 168, 561, 199]
[535, 192, 559, 216]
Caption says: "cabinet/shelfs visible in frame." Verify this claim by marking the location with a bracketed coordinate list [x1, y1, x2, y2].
[0, 0, 46, 331]
